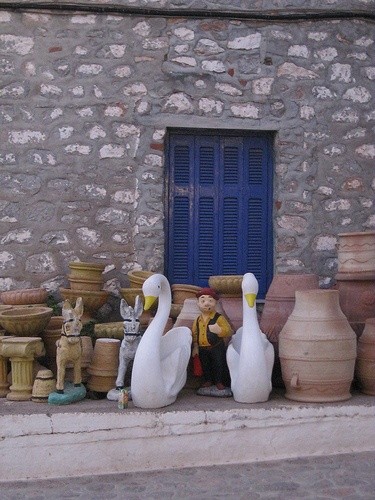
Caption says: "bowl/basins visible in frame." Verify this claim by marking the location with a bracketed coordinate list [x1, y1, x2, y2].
[60, 262, 110, 323]
[171, 284, 205, 305]
[118, 270, 159, 323]
[1, 285, 52, 338]
[207, 275, 244, 298]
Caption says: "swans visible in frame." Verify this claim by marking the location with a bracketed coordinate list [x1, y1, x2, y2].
[130, 273, 193, 409]
[226, 272, 275, 404]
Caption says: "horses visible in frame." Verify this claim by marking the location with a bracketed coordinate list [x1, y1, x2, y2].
[115, 295, 143, 390]
[56, 296, 84, 394]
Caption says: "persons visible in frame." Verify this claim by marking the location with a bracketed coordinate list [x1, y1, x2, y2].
[191, 290, 234, 389]
